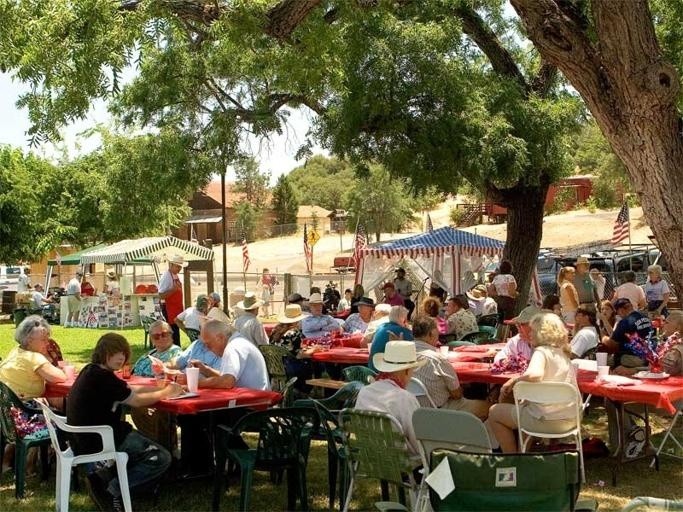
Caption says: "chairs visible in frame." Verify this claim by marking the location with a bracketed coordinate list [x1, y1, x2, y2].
[184, 327, 199, 343]
[1, 381, 61, 498]
[429, 448, 598, 511]
[313, 399, 347, 512]
[339, 408, 421, 512]
[258, 345, 297, 404]
[513, 381, 592, 485]
[213, 408, 319, 512]
[141, 315, 156, 349]
[33, 398, 132, 512]
[412, 407, 493, 511]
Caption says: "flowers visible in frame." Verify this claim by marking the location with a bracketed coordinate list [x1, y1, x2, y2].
[625, 316, 682, 368]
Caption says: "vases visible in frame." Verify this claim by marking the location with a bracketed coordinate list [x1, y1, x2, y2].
[648, 359, 664, 372]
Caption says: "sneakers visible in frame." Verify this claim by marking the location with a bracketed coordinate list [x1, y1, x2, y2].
[84, 471, 103, 498]
[92, 486, 122, 507]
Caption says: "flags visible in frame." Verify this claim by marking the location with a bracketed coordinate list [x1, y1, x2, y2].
[348, 216, 367, 267]
[302, 221, 313, 273]
[612, 198, 634, 247]
[240, 229, 251, 271]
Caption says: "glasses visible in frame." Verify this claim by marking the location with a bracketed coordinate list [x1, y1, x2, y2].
[152, 331, 173, 341]
[25, 318, 43, 335]
[76, 272, 82, 276]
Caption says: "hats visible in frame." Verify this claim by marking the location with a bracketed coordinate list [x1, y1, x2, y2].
[372, 341, 430, 373]
[237, 294, 264, 309]
[576, 256, 590, 265]
[286, 293, 302, 301]
[381, 282, 395, 290]
[613, 297, 630, 313]
[204, 308, 233, 324]
[276, 307, 306, 324]
[165, 255, 188, 267]
[107, 270, 115, 279]
[309, 292, 325, 305]
[395, 268, 406, 274]
[511, 305, 537, 324]
[466, 288, 485, 300]
[353, 297, 375, 307]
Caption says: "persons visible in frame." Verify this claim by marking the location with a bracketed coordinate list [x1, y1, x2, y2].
[133, 319, 185, 377]
[589, 268, 606, 300]
[457, 294, 479, 331]
[612, 310, 683, 461]
[429, 269, 448, 303]
[105, 271, 120, 293]
[149, 308, 222, 380]
[565, 303, 601, 359]
[360, 304, 391, 347]
[270, 304, 322, 393]
[487, 283, 504, 314]
[466, 290, 485, 316]
[422, 296, 448, 334]
[573, 257, 601, 312]
[232, 292, 270, 347]
[381, 282, 404, 305]
[597, 300, 619, 337]
[368, 306, 413, 373]
[189, 318, 272, 391]
[444, 298, 475, 340]
[476, 284, 498, 315]
[542, 294, 561, 312]
[289, 293, 306, 305]
[602, 297, 657, 370]
[301, 292, 339, 338]
[0, 314, 66, 476]
[493, 260, 517, 338]
[341, 297, 375, 335]
[611, 270, 647, 312]
[208, 292, 220, 306]
[159, 255, 189, 346]
[33, 284, 52, 308]
[355, 340, 423, 485]
[175, 294, 213, 336]
[392, 268, 415, 321]
[67, 333, 185, 510]
[351, 284, 374, 313]
[409, 316, 491, 421]
[643, 265, 671, 318]
[337, 289, 352, 312]
[18, 266, 32, 292]
[490, 314, 581, 454]
[257, 268, 277, 316]
[556, 266, 580, 323]
[65, 271, 83, 328]
[494, 305, 539, 370]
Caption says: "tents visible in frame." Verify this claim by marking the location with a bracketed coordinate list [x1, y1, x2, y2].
[81, 235, 214, 308]
[354, 226, 507, 298]
[45, 241, 152, 311]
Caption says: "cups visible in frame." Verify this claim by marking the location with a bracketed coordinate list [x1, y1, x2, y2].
[596, 353, 608, 366]
[598, 367, 609, 380]
[439, 346, 449, 361]
[185, 368, 200, 393]
